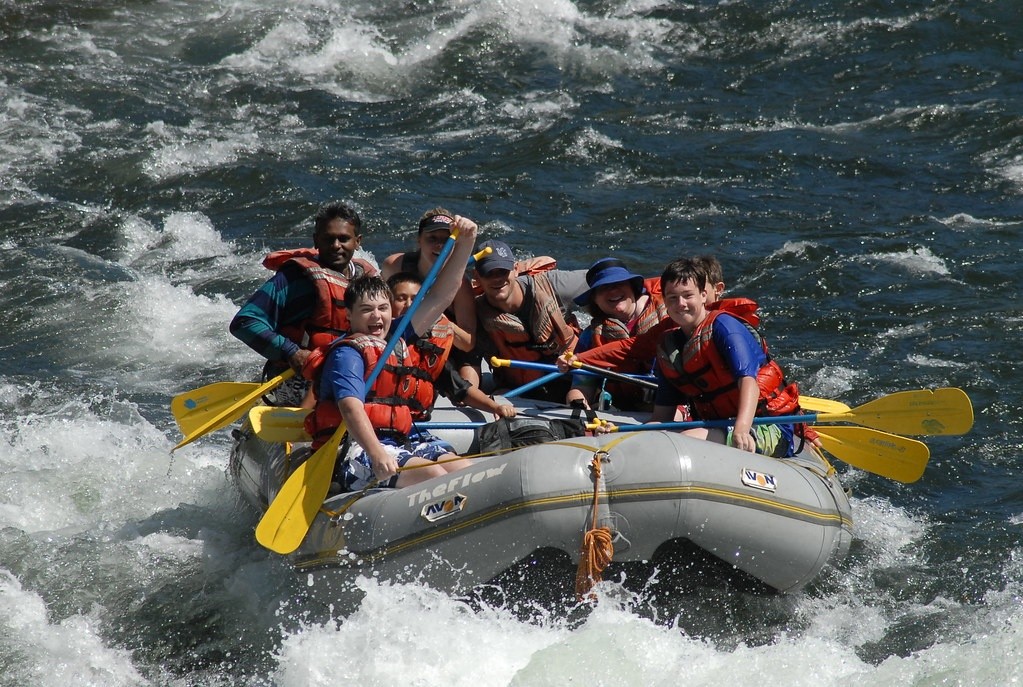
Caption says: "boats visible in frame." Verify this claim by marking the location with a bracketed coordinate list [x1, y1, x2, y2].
[227, 412, 854, 601]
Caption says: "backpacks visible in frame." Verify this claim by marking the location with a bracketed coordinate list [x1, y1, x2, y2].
[475, 416, 583, 455]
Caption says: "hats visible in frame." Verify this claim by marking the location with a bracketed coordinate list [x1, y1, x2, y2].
[572, 257, 647, 307]
[473, 239, 514, 277]
[417, 214, 454, 232]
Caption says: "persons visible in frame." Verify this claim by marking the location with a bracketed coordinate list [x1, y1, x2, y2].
[379, 206, 482, 406]
[650, 258, 796, 457]
[385, 270, 517, 419]
[228, 204, 376, 406]
[310, 213, 478, 491]
[560, 257, 669, 414]
[693, 254, 822, 447]
[472, 239, 592, 404]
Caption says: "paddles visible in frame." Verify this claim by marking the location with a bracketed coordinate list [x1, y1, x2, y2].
[254, 223, 460, 554]
[168, 241, 496, 455]
[582, 384, 976, 437]
[248, 403, 498, 445]
[489, 354, 856, 420]
[567, 356, 930, 485]
